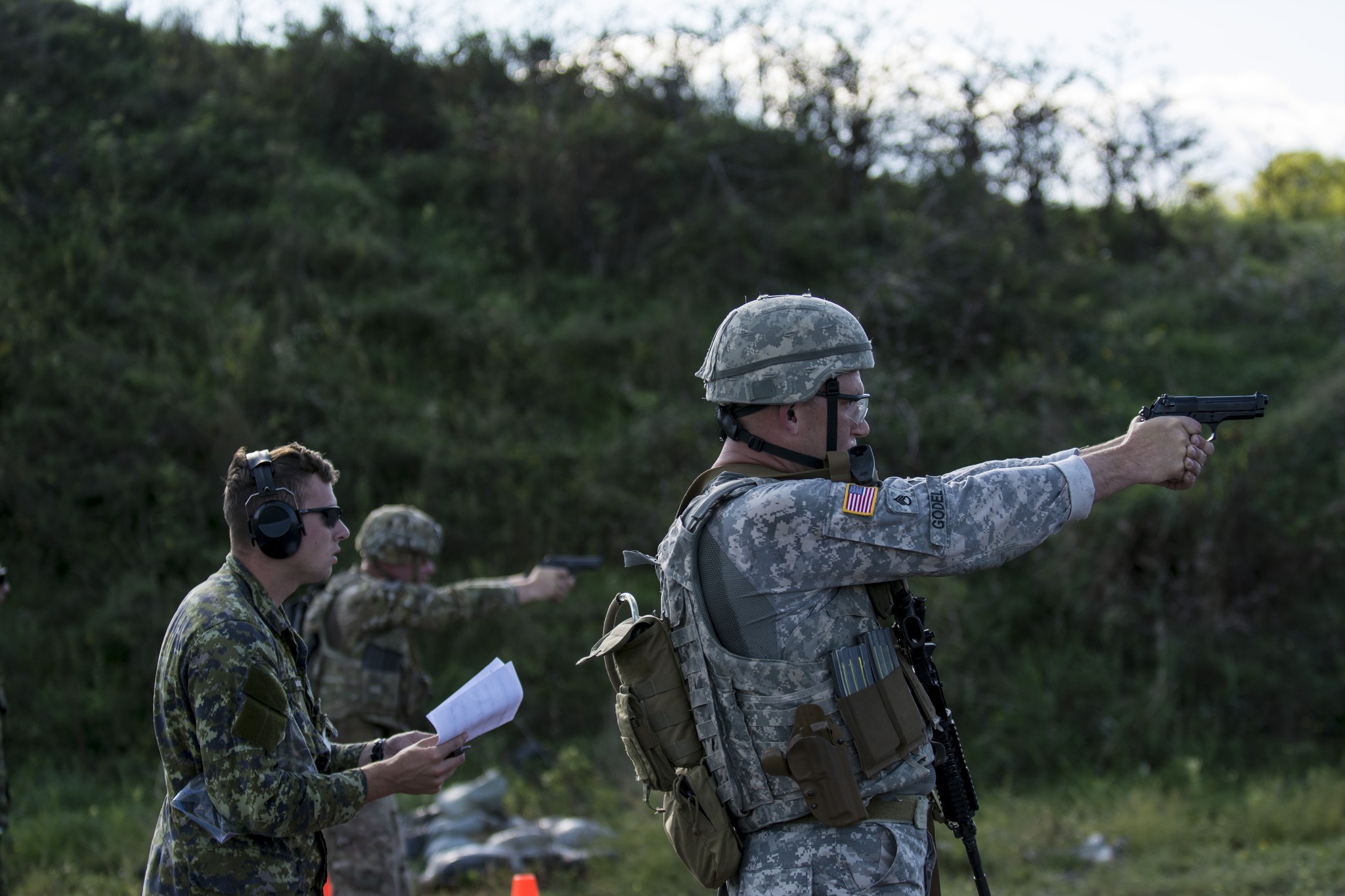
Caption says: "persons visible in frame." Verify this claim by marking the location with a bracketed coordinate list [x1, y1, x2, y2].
[137, 433, 468, 896]
[648, 288, 1214, 896]
[297, 502, 575, 894]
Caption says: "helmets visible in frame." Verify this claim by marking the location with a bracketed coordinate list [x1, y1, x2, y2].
[354, 504, 445, 563]
[704, 294, 874, 406]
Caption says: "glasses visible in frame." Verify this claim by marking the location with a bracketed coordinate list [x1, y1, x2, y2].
[811, 390, 871, 424]
[294, 505, 345, 529]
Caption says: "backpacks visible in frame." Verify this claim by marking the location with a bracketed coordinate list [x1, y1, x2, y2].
[588, 590, 742, 890]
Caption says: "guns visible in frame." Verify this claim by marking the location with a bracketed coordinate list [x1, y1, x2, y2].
[541, 554, 603, 576]
[1136, 392, 1269, 443]
[844, 442, 992, 896]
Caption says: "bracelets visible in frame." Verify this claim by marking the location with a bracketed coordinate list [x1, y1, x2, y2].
[370, 737, 386, 761]
